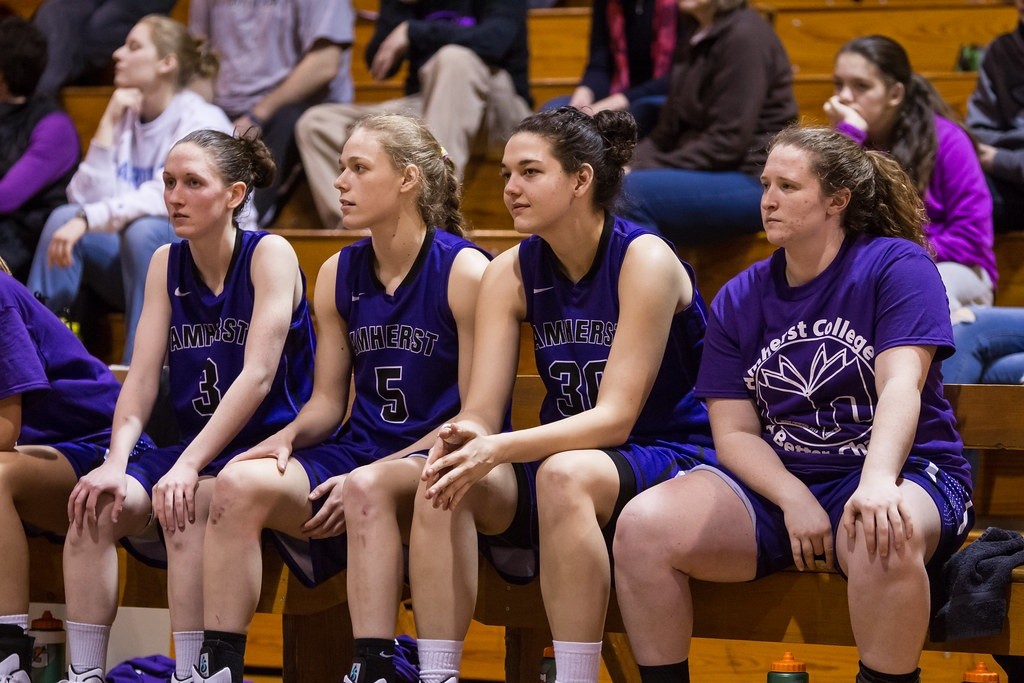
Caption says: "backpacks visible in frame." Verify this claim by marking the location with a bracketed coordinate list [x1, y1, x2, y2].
[105, 655, 176, 683]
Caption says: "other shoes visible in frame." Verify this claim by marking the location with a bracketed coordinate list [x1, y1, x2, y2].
[57, 664, 104, 683]
[343, 657, 387, 683]
[171, 664, 232, 683]
[0, 653, 33, 683]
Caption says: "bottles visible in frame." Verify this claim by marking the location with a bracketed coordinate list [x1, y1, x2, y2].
[28, 612, 66, 683]
[536, 646, 557, 683]
[767, 652, 809, 683]
[961, 662, 999, 683]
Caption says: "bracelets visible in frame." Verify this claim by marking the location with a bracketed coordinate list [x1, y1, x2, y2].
[244, 107, 274, 130]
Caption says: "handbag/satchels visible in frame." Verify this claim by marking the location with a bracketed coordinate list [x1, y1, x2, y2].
[36, 290, 111, 360]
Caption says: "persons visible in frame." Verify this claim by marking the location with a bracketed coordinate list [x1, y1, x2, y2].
[939, 305, 1024, 384]
[822, 34, 999, 307]
[539, 1, 688, 141]
[0, 0, 180, 285]
[291, 0, 536, 229]
[408, 106, 719, 683]
[963, 0, 1024, 230]
[186, 0, 356, 231]
[190, 113, 495, 683]
[611, 117, 975, 682]
[56, 127, 318, 683]
[25, 15, 257, 364]
[613, 0, 796, 237]
[0, 260, 158, 683]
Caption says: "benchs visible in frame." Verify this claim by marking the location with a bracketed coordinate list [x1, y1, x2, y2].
[0, 0, 1024, 655]
